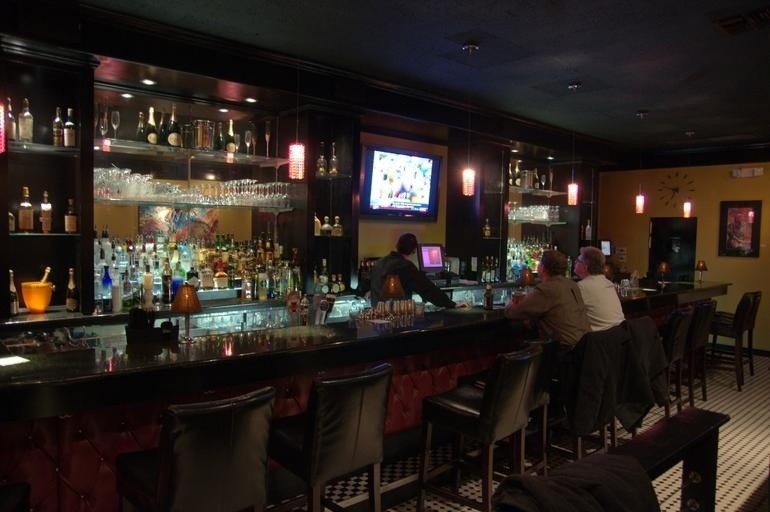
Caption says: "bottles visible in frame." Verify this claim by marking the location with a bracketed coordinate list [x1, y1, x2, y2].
[135, 103, 182, 148]
[5, 97, 18, 142]
[18, 187, 35, 233]
[225, 118, 236, 152]
[38, 190, 54, 233]
[64, 108, 77, 150]
[321, 258, 329, 275]
[619, 279, 631, 297]
[65, 267, 80, 312]
[534, 168, 541, 191]
[358, 261, 380, 278]
[329, 140, 340, 176]
[315, 140, 328, 177]
[94, 97, 108, 137]
[99, 329, 272, 375]
[9, 269, 19, 317]
[586, 219, 592, 240]
[507, 236, 572, 282]
[508, 162, 521, 186]
[41, 267, 53, 284]
[481, 256, 500, 282]
[484, 217, 491, 238]
[316, 273, 346, 295]
[315, 215, 344, 236]
[358, 298, 416, 319]
[483, 285, 494, 310]
[18, 98, 34, 145]
[63, 199, 78, 233]
[215, 121, 225, 152]
[94, 225, 301, 303]
[52, 106, 64, 147]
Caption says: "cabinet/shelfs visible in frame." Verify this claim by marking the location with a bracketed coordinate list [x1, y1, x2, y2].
[448, 138, 599, 280]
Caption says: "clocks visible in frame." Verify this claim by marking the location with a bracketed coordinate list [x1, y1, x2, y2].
[657, 172, 696, 210]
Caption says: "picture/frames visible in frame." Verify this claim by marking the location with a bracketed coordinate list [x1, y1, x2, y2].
[718, 200, 763, 258]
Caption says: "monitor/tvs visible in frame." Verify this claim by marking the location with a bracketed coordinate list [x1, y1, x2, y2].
[362, 144, 440, 219]
[418, 241, 453, 277]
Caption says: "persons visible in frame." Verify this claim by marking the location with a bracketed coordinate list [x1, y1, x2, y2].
[502, 250, 592, 355]
[573, 246, 627, 334]
[367, 232, 471, 314]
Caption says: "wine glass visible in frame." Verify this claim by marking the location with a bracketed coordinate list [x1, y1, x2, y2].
[110, 110, 122, 140]
[265, 120, 272, 161]
[508, 201, 561, 220]
[93, 167, 179, 208]
[179, 178, 291, 208]
[244, 130, 252, 157]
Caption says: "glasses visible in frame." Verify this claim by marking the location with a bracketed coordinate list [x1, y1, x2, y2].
[574, 256, 583, 263]
